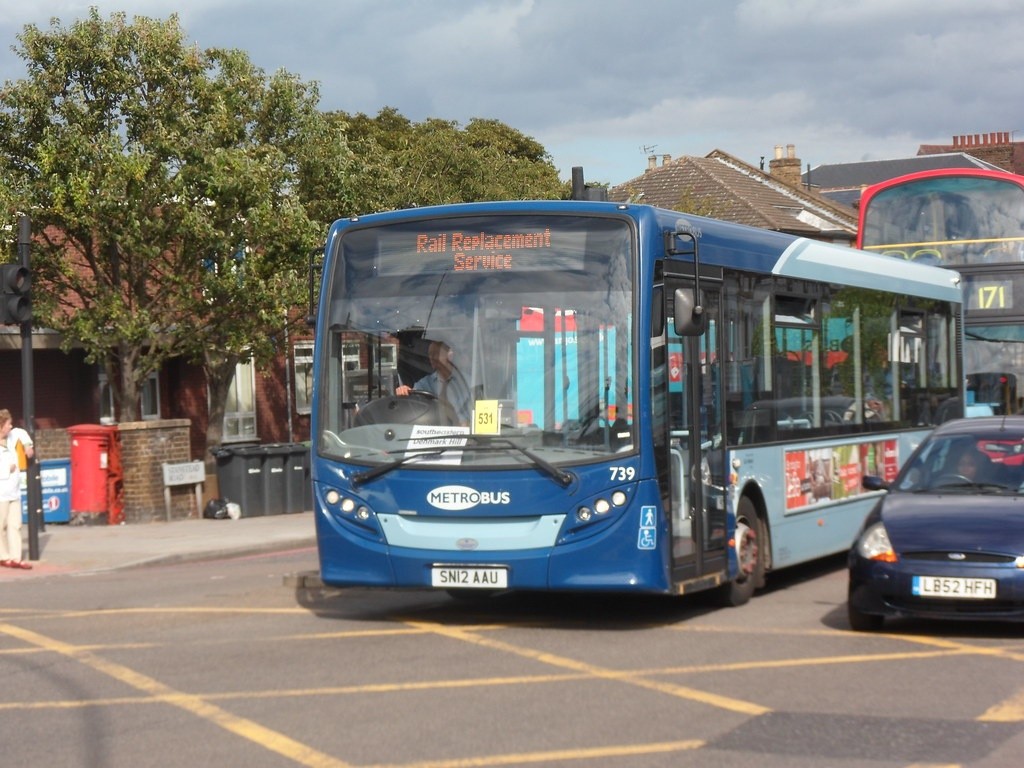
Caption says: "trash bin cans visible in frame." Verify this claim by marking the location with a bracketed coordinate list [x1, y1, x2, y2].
[65, 424, 114, 525]
[213, 442, 312, 519]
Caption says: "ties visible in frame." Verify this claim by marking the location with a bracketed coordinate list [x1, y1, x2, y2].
[439, 377, 454, 403]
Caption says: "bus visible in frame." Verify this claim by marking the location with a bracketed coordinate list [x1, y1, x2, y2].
[302, 196, 972, 609]
[849, 163, 1024, 422]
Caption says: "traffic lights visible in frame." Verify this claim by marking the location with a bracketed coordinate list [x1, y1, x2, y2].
[0, 262, 35, 327]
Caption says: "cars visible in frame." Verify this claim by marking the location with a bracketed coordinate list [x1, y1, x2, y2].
[846, 413, 1024, 632]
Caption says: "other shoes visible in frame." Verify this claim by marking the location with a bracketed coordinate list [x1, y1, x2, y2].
[9, 560, 33, 569]
[0, 560, 11, 568]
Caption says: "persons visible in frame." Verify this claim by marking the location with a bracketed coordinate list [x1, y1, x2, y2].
[396, 340, 473, 426]
[863, 340, 893, 424]
[955, 449, 979, 489]
[0, 409, 34, 570]
